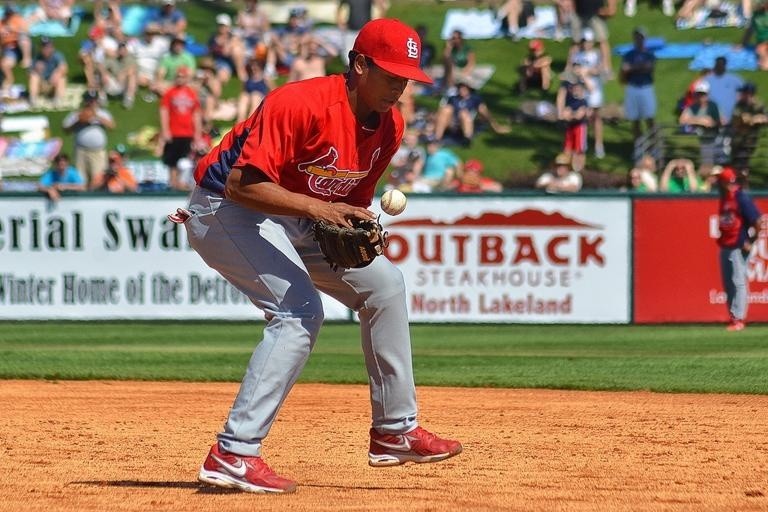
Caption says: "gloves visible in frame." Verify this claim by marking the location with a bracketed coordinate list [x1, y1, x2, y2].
[167, 208, 193, 224]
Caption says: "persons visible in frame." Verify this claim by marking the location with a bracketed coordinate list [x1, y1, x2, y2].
[184, 17, 464, 496]
[0, 1, 768, 196]
[712, 167, 764, 332]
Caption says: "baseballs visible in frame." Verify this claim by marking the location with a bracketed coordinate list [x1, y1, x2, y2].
[380, 191, 407, 214]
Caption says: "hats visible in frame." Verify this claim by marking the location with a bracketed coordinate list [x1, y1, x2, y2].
[720, 168, 738, 183]
[353, 18, 435, 87]
[529, 26, 756, 176]
[418, 132, 440, 143]
[408, 151, 423, 161]
[456, 78, 477, 90]
[4, 2, 309, 102]
[465, 160, 483, 172]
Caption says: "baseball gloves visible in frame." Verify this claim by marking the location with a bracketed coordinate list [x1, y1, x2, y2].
[316, 218, 384, 267]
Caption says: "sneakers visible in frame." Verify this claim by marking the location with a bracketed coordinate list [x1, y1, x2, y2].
[198, 445, 296, 495]
[726, 317, 744, 331]
[367, 425, 462, 467]
